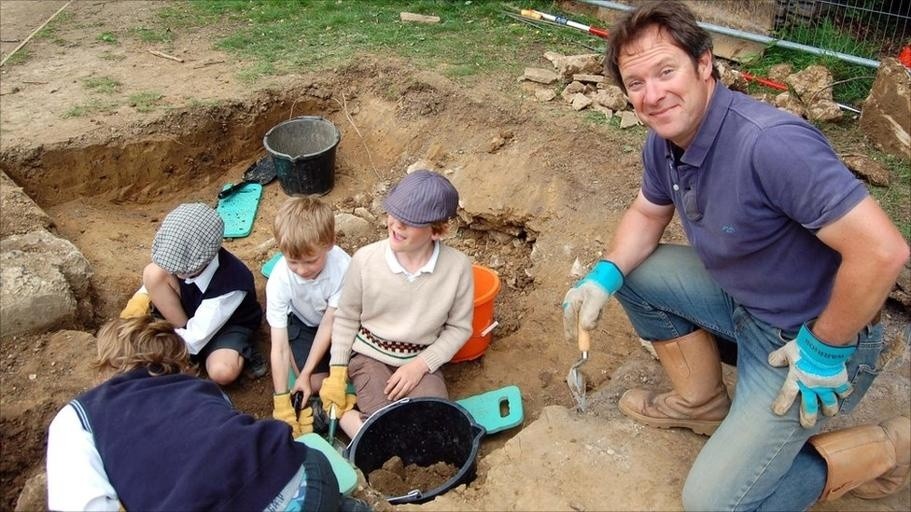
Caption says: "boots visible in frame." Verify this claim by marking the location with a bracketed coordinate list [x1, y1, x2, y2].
[617, 328, 731, 438]
[807, 415, 910, 502]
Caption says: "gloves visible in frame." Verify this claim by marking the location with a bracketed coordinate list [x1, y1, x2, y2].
[119, 292, 154, 319]
[563, 260, 624, 341]
[273, 390, 314, 439]
[319, 364, 358, 420]
[768, 317, 860, 428]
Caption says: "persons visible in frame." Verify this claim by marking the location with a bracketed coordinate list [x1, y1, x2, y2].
[43, 315, 372, 512]
[561, 3, 911, 510]
[322, 169, 475, 443]
[116, 203, 267, 387]
[261, 197, 354, 440]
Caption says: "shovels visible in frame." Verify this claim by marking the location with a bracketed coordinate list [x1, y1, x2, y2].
[218, 154, 277, 198]
[566, 311, 591, 411]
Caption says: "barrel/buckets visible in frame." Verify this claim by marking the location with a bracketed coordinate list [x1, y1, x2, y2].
[448, 262, 502, 364]
[262, 115, 342, 199]
[344, 394, 487, 506]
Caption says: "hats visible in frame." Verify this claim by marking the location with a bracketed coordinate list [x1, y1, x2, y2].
[382, 170, 458, 229]
[151, 201, 224, 275]
